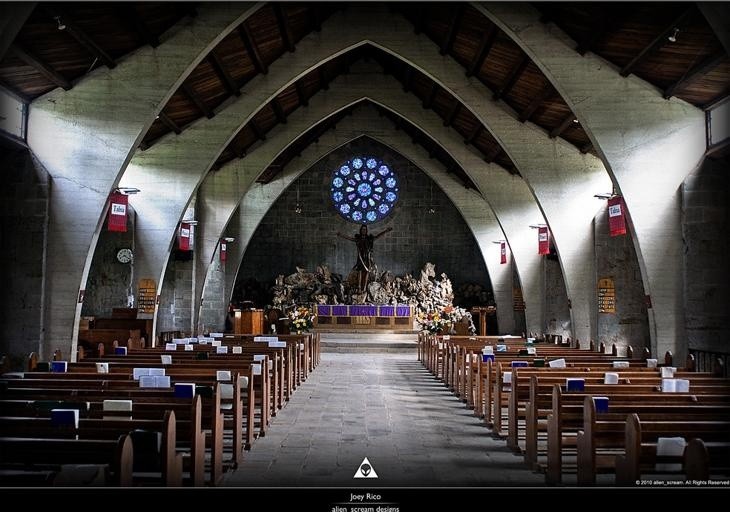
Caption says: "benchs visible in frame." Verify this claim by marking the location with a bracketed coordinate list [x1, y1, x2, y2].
[1, 326, 322, 485]
[416, 327, 730, 484]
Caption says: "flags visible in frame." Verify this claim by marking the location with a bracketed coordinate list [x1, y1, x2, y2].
[539, 227, 549, 254]
[501, 242, 506, 263]
[220, 239, 227, 261]
[180, 223, 190, 250]
[108, 193, 128, 232]
[608, 197, 626, 237]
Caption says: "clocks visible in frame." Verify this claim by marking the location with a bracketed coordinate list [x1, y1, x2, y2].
[117, 247, 133, 264]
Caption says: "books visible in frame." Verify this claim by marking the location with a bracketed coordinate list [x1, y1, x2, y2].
[2, 333, 304, 440]
[431, 335, 690, 470]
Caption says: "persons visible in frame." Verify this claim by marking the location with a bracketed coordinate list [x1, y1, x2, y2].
[336, 224, 393, 295]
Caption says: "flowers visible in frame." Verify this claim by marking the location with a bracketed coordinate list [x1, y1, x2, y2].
[290, 305, 319, 336]
[418, 304, 452, 336]
